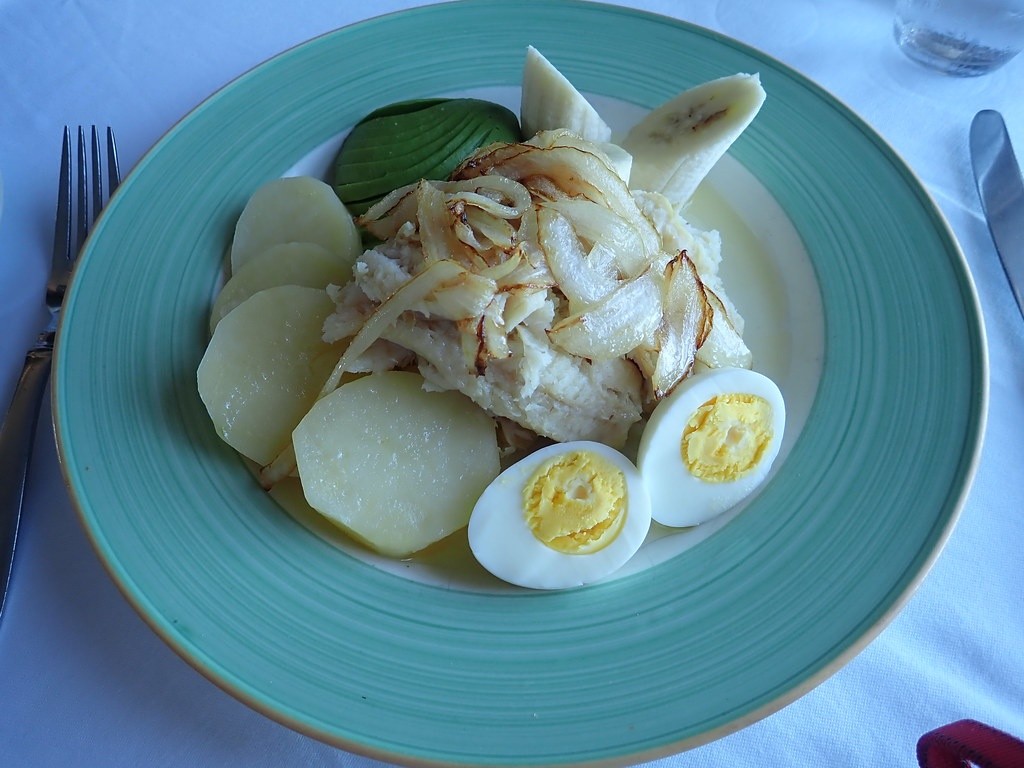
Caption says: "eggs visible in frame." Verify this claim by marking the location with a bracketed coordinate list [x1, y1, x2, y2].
[469, 440, 653, 591]
[638, 369, 787, 528]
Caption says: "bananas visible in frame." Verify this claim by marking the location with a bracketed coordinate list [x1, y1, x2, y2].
[521, 45, 768, 217]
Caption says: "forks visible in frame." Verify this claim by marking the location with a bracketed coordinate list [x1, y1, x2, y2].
[0, 123, 123, 619]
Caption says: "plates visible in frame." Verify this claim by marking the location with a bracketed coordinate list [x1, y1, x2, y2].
[51, 0, 992, 767]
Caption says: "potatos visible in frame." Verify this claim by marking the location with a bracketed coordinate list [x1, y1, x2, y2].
[197, 176, 502, 560]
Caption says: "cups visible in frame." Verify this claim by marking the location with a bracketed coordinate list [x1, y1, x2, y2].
[887, 0, 1024, 79]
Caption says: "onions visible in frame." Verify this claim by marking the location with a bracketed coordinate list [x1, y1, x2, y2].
[260, 128, 751, 495]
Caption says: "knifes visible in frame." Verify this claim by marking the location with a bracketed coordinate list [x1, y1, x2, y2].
[967, 110, 1024, 326]
